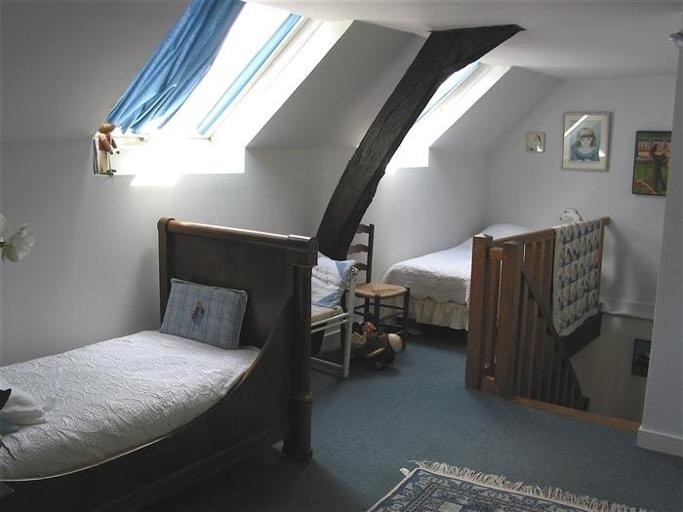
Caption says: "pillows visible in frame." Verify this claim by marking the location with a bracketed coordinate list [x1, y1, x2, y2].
[158, 276, 248, 348]
[310, 252, 356, 311]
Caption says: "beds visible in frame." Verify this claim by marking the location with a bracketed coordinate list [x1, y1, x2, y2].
[383, 224, 533, 344]
[0, 218, 319, 511]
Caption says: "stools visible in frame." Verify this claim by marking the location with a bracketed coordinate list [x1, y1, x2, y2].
[310, 251, 359, 379]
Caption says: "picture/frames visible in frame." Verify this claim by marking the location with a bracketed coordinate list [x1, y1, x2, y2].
[629, 338, 651, 378]
[631, 131, 672, 196]
[561, 111, 614, 173]
[526, 132, 546, 153]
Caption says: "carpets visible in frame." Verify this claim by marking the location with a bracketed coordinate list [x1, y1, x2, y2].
[366, 460, 648, 512]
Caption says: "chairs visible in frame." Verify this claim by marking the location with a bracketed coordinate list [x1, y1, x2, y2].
[346, 224, 411, 348]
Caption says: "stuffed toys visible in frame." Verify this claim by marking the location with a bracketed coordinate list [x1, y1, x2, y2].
[352, 321, 403, 372]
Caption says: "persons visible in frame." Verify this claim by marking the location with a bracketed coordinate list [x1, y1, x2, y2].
[98, 123, 120, 175]
[649, 143, 669, 190]
[189, 300, 204, 342]
[534, 135, 542, 145]
[571, 127, 599, 162]
